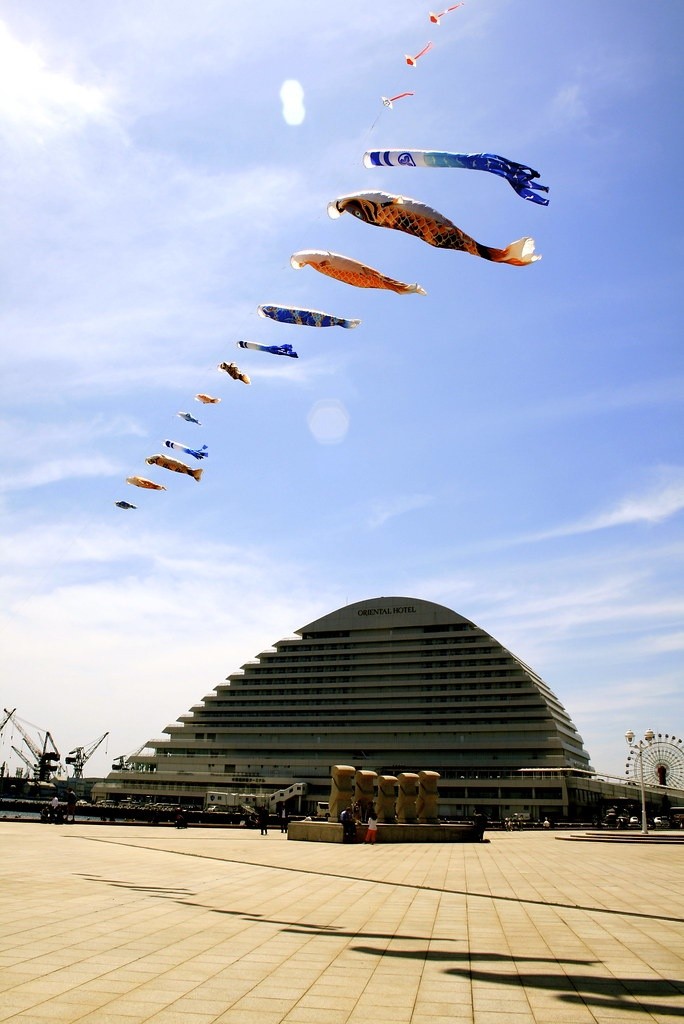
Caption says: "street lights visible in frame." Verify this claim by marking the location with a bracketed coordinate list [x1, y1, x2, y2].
[626, 728, 657, 834]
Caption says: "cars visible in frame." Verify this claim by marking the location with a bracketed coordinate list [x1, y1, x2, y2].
[76, 799, 221, 811]
[607, 807, 684, 827]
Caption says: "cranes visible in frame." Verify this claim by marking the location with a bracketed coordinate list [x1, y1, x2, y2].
[0, 708, 70, 780]
[65, 730, 108, 777]
[114, 741, 147, 771]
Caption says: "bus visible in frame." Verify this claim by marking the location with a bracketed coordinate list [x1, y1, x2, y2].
[316, 801, 330, 817]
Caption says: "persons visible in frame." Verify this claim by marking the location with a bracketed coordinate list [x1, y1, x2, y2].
[184, 809, 192, 829]
[258, 805, 269, 835]
[358, 797, 373, 823]
[304, 814, 312, 821]
[548, 818, 555, 828]
[543, 819, 550, 829]
[279, 805, 289, 833]
[339, 807, 357, 837]
[505, 814, 524, 831]
[362, 812, 378, 844]
[65, 787, 79, 822]
[351, 809, 362, 825]
[247, 812, 258, 825]
[176, 813, 184, 829]
[42, 806, 49, 822]
[50, 793, 58, 821]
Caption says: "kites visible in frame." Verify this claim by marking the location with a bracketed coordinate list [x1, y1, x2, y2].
[111, 0, 549, 517]
